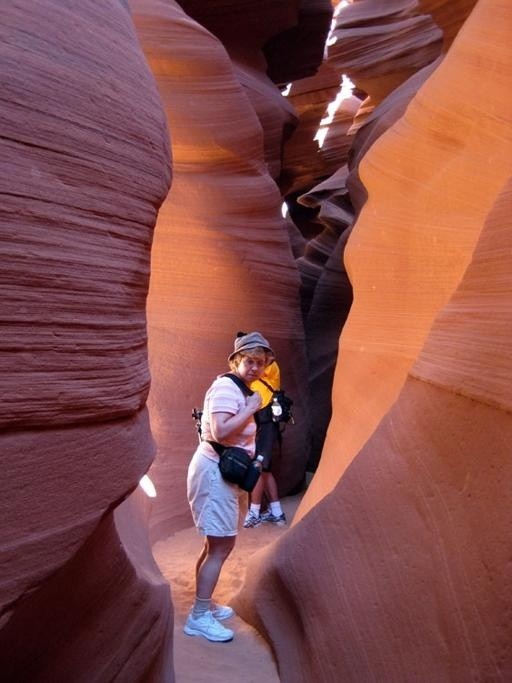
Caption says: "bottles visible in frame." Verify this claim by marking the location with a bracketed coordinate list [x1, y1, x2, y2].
[271, 398, 283, 421]
[252, 455, 265, 473]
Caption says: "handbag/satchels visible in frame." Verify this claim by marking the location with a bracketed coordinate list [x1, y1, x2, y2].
[260, 379, 294, 425]
[209, 440, 251, 493]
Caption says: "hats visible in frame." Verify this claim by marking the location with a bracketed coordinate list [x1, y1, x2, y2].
[228, 332, 276, 367]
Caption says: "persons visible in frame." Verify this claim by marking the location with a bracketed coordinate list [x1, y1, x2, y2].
[239, 345, 288, 530]
[180, 329, 277, 644]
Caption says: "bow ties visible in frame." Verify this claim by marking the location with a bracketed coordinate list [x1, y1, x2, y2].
[243, 511, 287, 528]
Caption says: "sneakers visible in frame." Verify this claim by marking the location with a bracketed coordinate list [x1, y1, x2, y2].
[183, 605, 235, 642]
